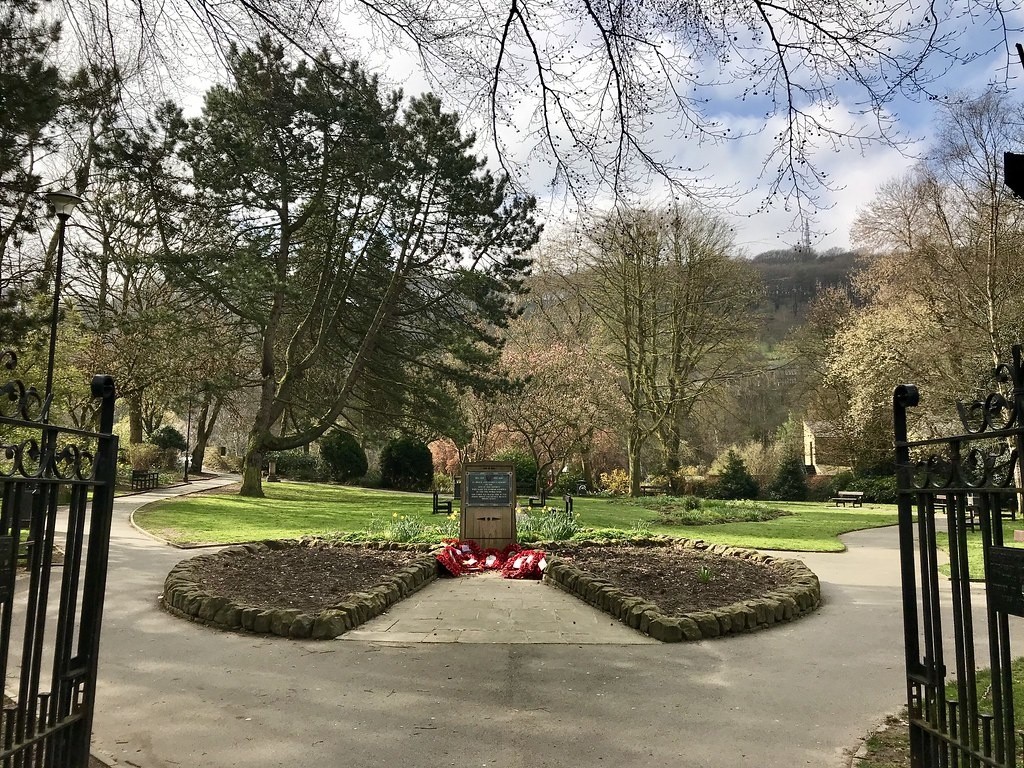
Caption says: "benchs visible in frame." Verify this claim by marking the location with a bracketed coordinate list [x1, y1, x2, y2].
[433, 491, 452, 515]
[641, 486, 672, 498]
[934, 495, 957, 515]
[1001, 500, 1016, 521]
[832, 491, 864, 508]
[528, 487, 545, 507]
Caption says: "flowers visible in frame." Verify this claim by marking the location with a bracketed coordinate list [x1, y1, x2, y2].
[458, 539, 485, 572]
[504, 543, 522, 566]
[436, 555, 459, 578]
[501, 551, 547, 579]
[482, 548, 505, 570]
[444, 546, 470, 574]
